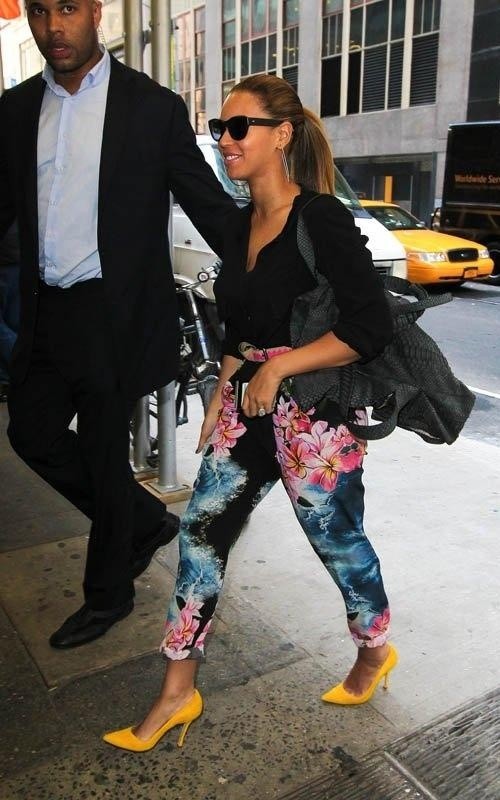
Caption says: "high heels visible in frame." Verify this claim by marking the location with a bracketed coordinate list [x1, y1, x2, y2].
[321, 643, 398, 705]
[103, 689, 202, 753]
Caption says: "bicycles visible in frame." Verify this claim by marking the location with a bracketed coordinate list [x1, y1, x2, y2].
[150, 262, 222, 449]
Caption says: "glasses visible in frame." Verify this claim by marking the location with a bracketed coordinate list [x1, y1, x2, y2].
[208, 115, 284, 141]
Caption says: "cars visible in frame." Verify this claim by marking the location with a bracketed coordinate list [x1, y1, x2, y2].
[358, 199, 494, 291]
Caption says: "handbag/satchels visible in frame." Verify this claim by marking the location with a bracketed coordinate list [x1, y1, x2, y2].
[291, 275, 476, 445]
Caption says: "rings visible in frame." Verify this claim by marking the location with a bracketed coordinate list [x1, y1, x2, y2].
[256, 409, 266, 418]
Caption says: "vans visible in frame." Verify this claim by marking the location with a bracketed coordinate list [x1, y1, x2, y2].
[169, 133, 408, 379]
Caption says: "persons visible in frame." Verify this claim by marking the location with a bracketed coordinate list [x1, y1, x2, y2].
[101, 74, 399, 751]
[0, 1, 241, 652]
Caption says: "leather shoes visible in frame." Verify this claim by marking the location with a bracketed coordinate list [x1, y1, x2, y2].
[133, 511, 180, 579]
[48, 599, 134, 649]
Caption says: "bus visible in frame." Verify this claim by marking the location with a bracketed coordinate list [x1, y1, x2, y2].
[441, 121, 499, 278]
